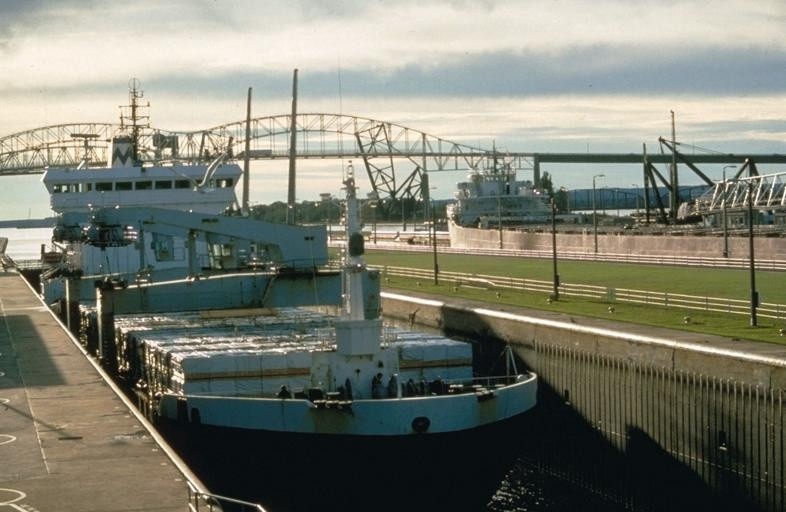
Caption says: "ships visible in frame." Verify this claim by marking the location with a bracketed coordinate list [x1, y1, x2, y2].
[12, 130, 540, 493]
[444, 136, 786, 270]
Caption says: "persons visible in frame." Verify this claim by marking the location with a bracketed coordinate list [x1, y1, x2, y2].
[277, 385, 292, 399]
[372, 372, 446, 399]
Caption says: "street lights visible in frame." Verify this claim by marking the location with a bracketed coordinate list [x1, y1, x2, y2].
[287, 185, 438, 288]
[529, 187, 561, 304]
[591, 172, 606, 251]
[720, 161, 738, 257]
[722, 176, 759, 330]
